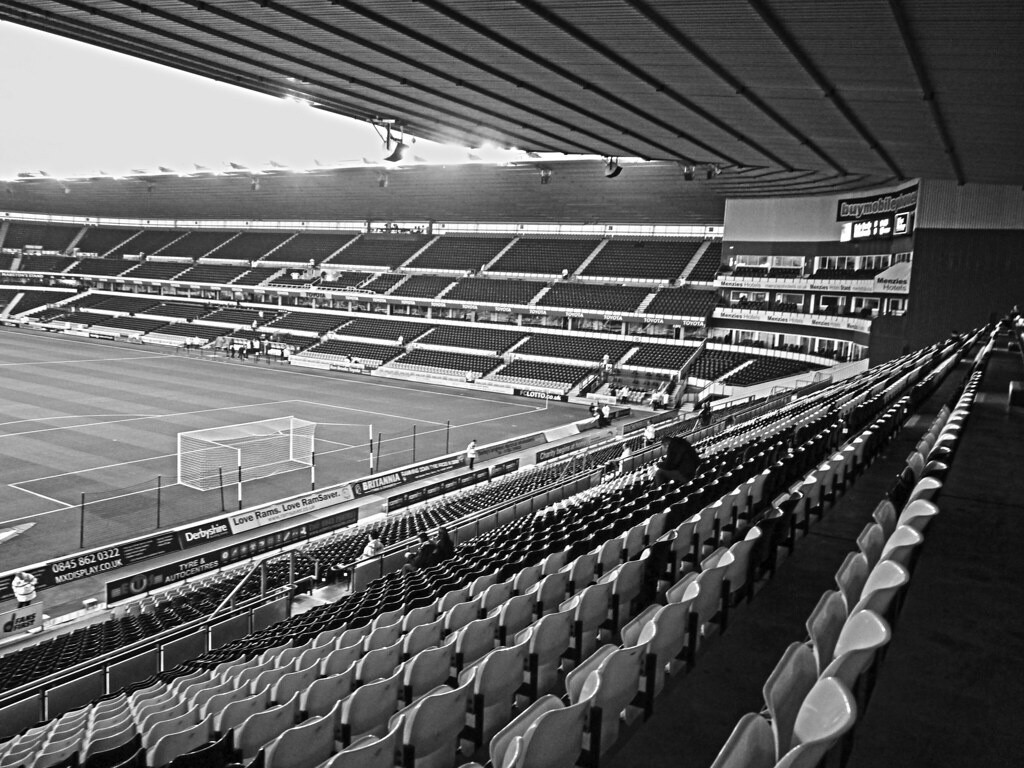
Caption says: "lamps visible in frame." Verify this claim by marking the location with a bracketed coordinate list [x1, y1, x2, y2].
[251, 176, 259, 191]
[378, 173, 389, 189]
[683, 164, 696, 181]
[602, 155, 622, 178]
[707, 161, 722, 180]
[538, 163, 553, 185]
[370, 118, 409, 162]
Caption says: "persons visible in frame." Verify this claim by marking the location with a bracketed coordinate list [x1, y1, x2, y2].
[398, 335, 403, 345]
[467, 440, 476, 470]
[348, 530, 384, 563]
[176, 333, 286, 366]
[644, 419, 655, 446]
[650, 389, 669, 410]
[563, 267, 568, 279]
[402, 527, 454, 575]
[1001, 305, 1021, 326]
[611, 384, 629, 404]
[21, 315, 30, 326]
[590, 400, 610, 429]
[619, 443, 632, 472]
[480, 264, 485, 277]
[692, 401, 711, 432]
[259, 310, 264, 319]
[11, 571, 38, 608]
[252, 318, 257, 332]
[466, 370, 474, 383]
[936, 329, 961, 356]
[236, 299, 241, 308]
[345, 354, 359, 363]
[603, 353, 609, 367]
[653, 436, 701, 487]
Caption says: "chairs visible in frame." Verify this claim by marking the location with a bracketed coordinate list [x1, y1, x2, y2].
[0, 304, 1024, 767]
